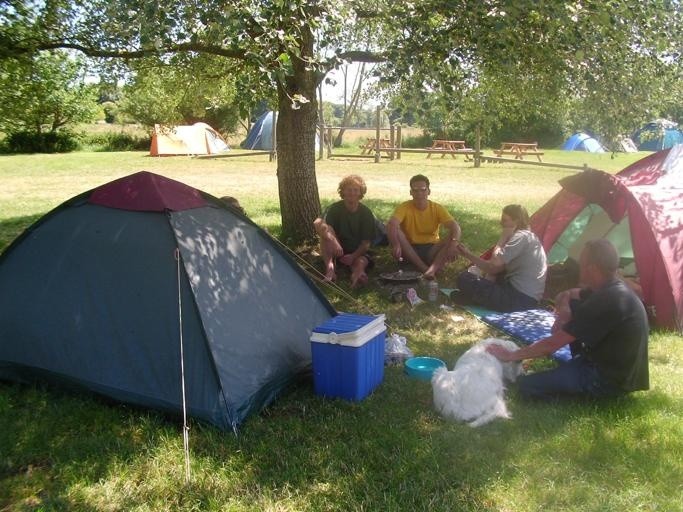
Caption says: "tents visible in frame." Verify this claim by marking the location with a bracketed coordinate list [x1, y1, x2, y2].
[0, 171, 343, 436]
[614, 138, 638, 153]
[241, 110, 327, 153]
[149, 121, 220, 157]
[629, 119, 683, 151]
[467, 144, 683, 334]
[191, 122, 232, 152]
[560, 132, 605, 153]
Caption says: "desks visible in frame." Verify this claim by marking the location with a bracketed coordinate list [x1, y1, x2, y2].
[360, 138, 397, 160]
[427, 140, 469, 160]
[493, 143, 543, 164]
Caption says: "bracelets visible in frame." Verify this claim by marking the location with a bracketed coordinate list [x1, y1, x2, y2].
[448, 237, 460, 243]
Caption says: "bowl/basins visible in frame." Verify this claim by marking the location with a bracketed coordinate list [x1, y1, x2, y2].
[404, 356, 446, 380]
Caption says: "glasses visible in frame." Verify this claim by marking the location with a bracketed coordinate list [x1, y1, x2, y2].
[412, 186, 427, 191]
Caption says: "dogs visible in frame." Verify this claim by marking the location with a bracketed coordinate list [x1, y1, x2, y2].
[431, 338, 527, 428]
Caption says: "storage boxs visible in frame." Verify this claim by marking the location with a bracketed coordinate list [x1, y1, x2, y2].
[310, 313, 388, 404]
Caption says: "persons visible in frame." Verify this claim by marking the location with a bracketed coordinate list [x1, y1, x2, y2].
[450, 205, 548, 311]
[386, 174, 462, 288]
[220, 195, 244, 214]
[485, 239, 650, 399]
[313, 175, 377, 284]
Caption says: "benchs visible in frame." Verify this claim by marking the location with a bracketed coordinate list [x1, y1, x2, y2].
[380, 146, 397, 159]
[493, 150, 528, 160]
[520, 151, 544, 162]
[451, 149, 472, 159]
[359, 145, 375, 155]
[426, 147, 447, 158]
